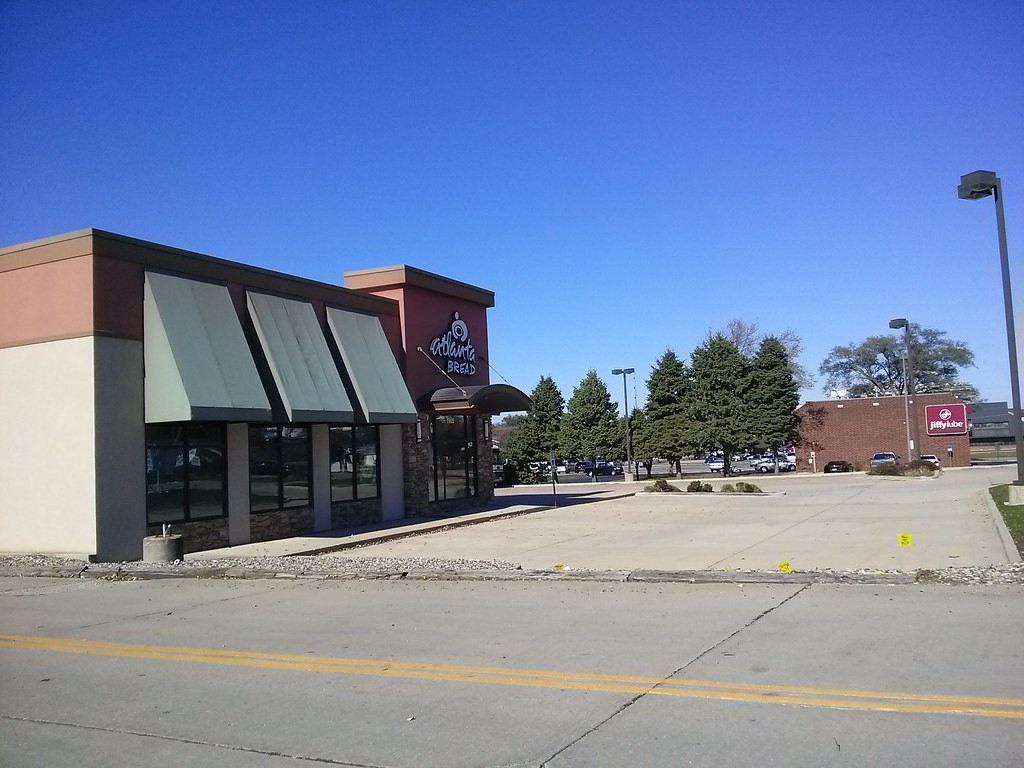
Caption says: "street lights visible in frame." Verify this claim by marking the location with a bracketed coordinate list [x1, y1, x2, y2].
[888, 318, 921, 460]
[612, 367, 635, 473]
[959, 170, 1023, 485]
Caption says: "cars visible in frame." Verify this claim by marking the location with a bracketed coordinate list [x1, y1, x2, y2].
[706, 451, 796, 474]
[823, 460, 852, 473]
[919, 454, 941, 467]
[529, 456, 616, 474]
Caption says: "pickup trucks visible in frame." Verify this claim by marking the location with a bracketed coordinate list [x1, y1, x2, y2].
[868, 452, 902, 466]
[585, 462, 623, 477]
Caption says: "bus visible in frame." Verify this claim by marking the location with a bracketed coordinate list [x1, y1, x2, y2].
[492, 445, 509, 485]
